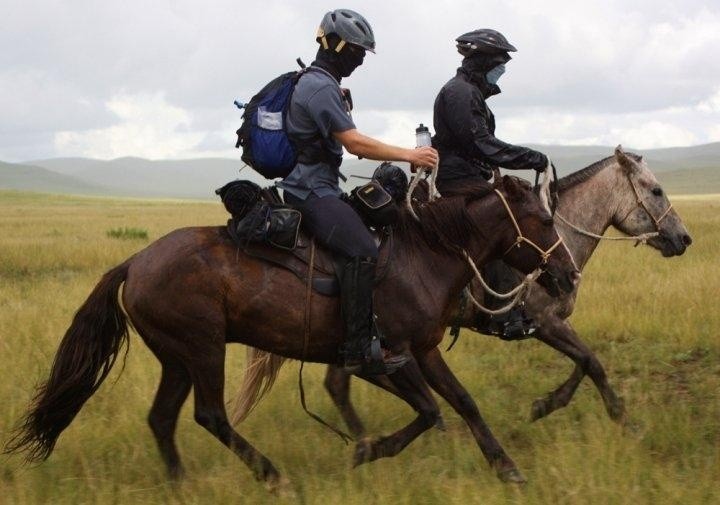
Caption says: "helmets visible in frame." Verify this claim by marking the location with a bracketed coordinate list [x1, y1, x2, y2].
[456, 29, 517, 56]
[317, 10, 375, 53]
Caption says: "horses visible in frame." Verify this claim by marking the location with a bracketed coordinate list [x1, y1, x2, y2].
[228, 144, 692, 446]
[0, 162, 581, 496]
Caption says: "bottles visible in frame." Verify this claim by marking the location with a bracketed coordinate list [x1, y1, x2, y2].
[416, 124, 432, 147]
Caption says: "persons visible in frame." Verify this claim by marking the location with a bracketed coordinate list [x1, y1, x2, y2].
[274, 7, 440, 376]
[432, 27, 553, 340]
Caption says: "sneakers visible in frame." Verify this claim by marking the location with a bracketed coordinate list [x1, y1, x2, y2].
[502, 319, 542, 339]
[343, 343, 412, 376]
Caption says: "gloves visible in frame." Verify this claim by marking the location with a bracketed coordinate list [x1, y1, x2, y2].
[534, 153, 549, 173]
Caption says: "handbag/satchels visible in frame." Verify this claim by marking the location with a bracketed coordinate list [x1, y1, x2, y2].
[265, 210, 302, 250]
[354, 180, 397, 229]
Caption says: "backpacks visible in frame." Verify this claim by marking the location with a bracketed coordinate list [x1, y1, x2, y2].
[238, 68, 335, 179]
